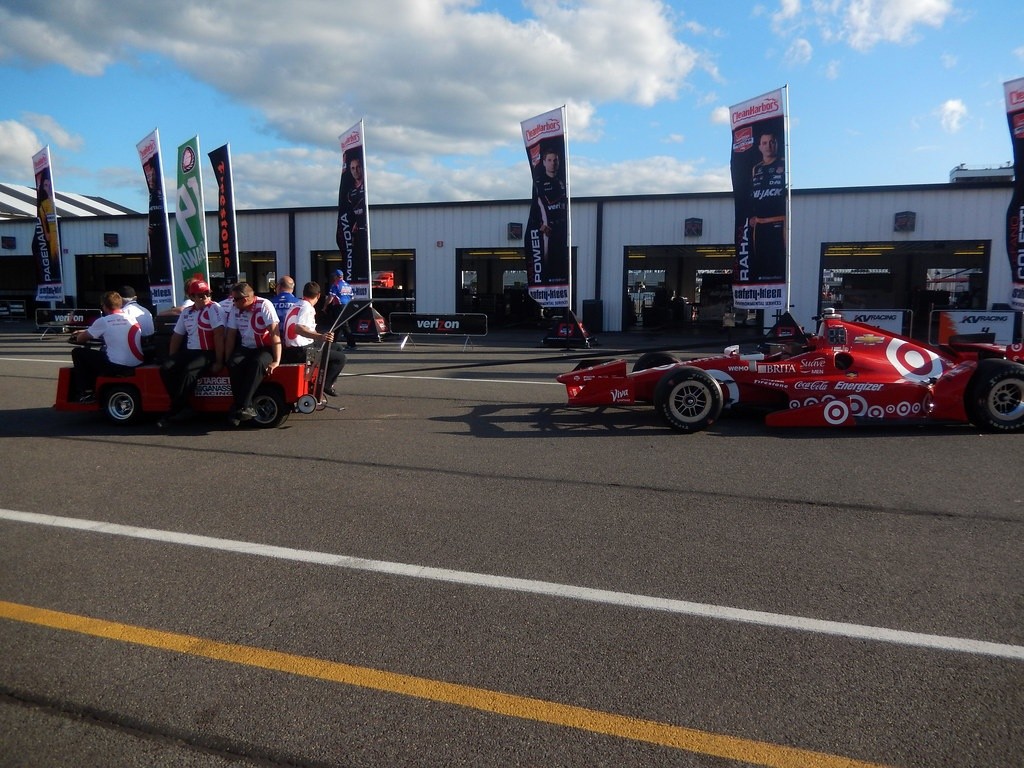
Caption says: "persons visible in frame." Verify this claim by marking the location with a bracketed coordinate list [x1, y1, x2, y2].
[169, 277, 282, 427]
[158, 281, 227, 428]
[347, 158, 368, 247]
[270, 276, 300, 337]
[119, 286, 154, 336]
[146, 164, 161, 265]
[533, 150, 568, 261]
[325, 269, 358, 351]
[749, 129, 786, 259]
[285, 282, 347, 397]
[40, 177, 59, 265]
[71, 291, 144, 403]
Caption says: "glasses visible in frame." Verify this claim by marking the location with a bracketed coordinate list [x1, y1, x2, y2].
[198, 292, 210, 298]
[234, 296, 248, 301]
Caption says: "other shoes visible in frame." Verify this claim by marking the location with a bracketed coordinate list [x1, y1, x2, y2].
[229, 416, 240, 426]
[239, 407, 257, 420]
[155, 419, 169, 428]
[324, 386, 337, 397]
[342, 345, 356, 352]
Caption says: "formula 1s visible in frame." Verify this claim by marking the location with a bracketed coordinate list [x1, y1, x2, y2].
[555, 309, 1024, 432]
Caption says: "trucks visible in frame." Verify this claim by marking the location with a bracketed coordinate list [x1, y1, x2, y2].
[699, 272, 748, 324]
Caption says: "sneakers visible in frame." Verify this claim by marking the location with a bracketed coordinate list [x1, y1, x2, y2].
[79, 395, 96, 404]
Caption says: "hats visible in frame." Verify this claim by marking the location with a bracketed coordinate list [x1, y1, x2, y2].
[331, 269, 343, 276]
[188, 281, 209, 296]
[119, 286, 136, 298]
